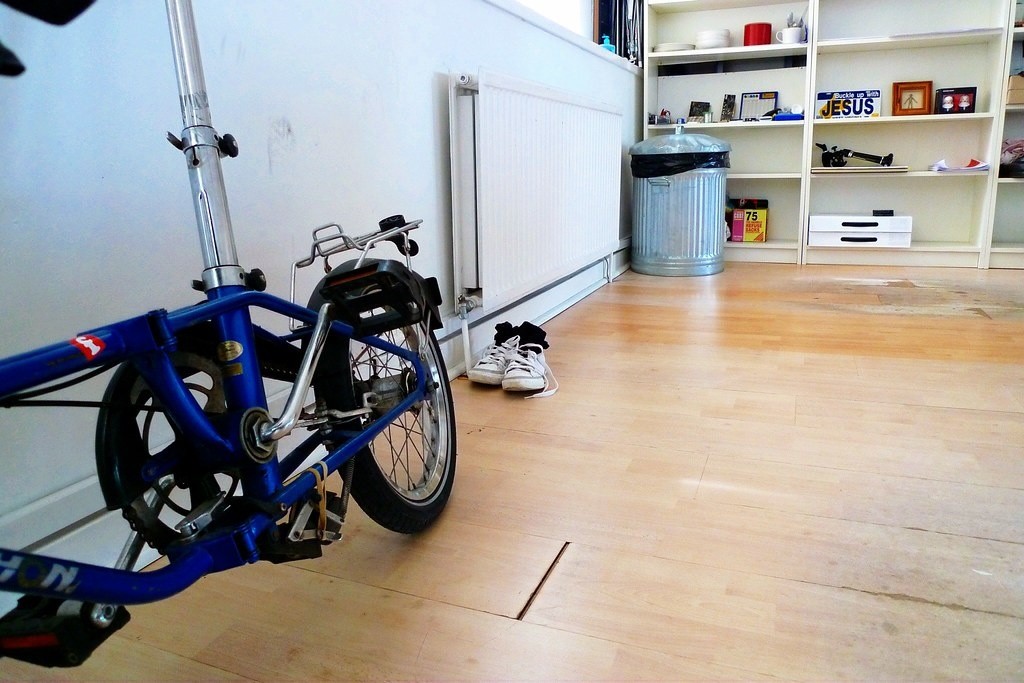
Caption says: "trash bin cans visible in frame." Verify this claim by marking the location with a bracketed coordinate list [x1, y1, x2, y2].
[628, 134, 733, 278]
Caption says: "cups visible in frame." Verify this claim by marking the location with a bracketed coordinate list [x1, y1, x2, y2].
[744, 23, 771, 45]
[697, 30, 730, 47]
[776, 27, 802, 43]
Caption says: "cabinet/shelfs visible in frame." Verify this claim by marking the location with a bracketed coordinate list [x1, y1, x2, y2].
[987, 0, 1024, 270]
[802, 0, 1002, 269]
[639, 0, 813, 270]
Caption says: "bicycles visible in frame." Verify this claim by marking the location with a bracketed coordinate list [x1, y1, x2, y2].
[0, 1, 457, 669]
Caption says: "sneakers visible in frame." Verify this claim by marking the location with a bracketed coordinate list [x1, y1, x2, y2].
[502, 343, 559, 399]
[468, 336, 520, 385]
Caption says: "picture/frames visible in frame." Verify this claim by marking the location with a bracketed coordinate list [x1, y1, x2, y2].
[934, 87, 978, 114]
[891, 81, 932, 116]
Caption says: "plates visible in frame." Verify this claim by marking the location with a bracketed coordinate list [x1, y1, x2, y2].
[653, 43, 695, 50]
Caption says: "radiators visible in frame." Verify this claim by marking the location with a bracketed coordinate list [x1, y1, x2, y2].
[446, 68, 626, 382]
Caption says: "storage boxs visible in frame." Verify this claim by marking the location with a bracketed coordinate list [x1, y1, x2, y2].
[730, 210, 745, 242]
[1006, 90, 1024, 104]
[809, 215, 913, 233]
[807, 232, 912, 250]
[1008, 76, 1024, 90]
[743, 209, 768, 242]
